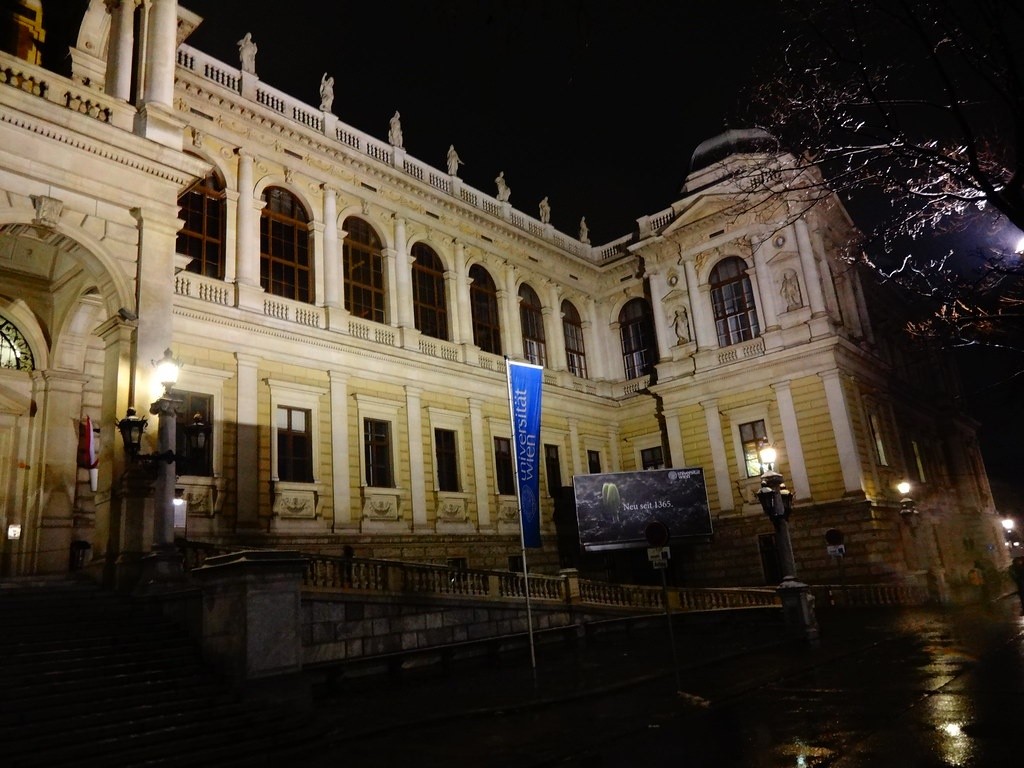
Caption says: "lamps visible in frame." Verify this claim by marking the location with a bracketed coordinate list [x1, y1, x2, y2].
[114, 407, 174, 465]
[895, 480, 922, 537]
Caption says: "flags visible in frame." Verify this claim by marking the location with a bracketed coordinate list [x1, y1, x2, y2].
[85, 418, 98, 491]
[507, 364, 548, 553]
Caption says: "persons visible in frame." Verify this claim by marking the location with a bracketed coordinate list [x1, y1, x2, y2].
[1008, 557, 1024, 616]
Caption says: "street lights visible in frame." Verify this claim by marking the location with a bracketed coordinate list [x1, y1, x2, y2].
[743, 433, 828, 653]
[151, 345, 181, 563]
